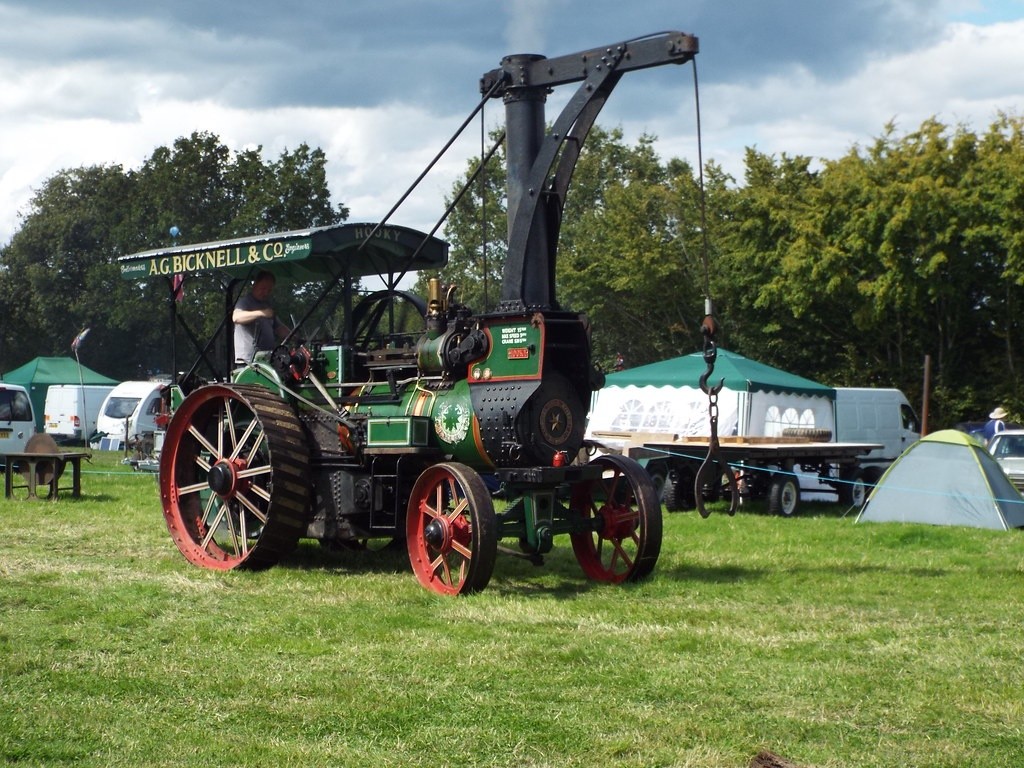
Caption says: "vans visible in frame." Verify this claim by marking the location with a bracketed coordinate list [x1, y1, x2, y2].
[43, 383, 116, 446]
[0, 383, 36, 455]
[833, 386, 923, 469]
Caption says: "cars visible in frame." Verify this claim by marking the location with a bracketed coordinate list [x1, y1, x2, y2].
[989, 428, 1023, 492]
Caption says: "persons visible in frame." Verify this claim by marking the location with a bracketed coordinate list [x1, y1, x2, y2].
[983, 407, 1006, 446]
[233, 270, 293, 373]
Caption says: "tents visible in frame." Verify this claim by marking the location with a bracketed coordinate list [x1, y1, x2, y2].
[0, 356, 122, 444]
[583, 347, 837, 480]
[853, 427, 1024, 531]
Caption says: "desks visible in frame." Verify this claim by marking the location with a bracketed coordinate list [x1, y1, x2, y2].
[0, 433, 87, 499]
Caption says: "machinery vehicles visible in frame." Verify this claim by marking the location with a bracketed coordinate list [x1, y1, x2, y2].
[116, 30, 741, 597]
